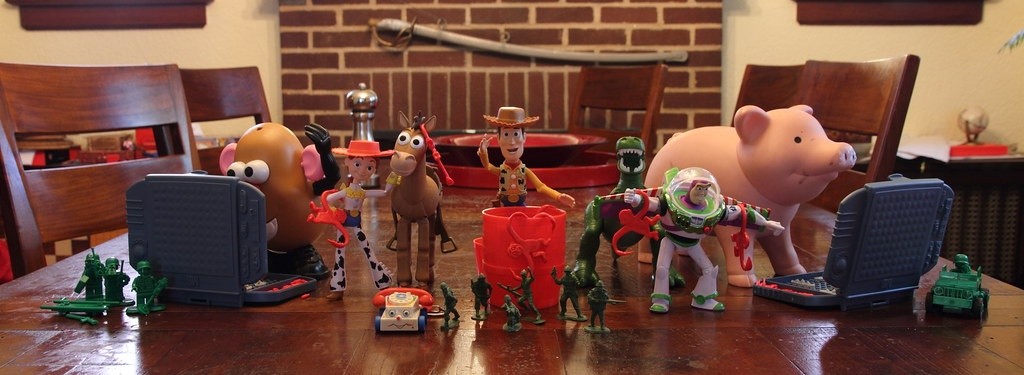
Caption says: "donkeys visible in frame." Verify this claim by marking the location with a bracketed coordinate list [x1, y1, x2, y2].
[388, 110, 444, 284]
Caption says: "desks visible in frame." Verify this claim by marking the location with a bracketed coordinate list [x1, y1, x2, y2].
[0, 186, 1023, 374]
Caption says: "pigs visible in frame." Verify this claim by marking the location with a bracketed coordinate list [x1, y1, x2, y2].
[637, 104, 857, 290]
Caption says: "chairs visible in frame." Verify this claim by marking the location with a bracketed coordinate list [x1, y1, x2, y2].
[794, 54, 922, 214]
[1, 61, 204, 279]
[152, 65, 271, 175]
[566, 63, 669, 160]
[732, 63, 871, 145]
